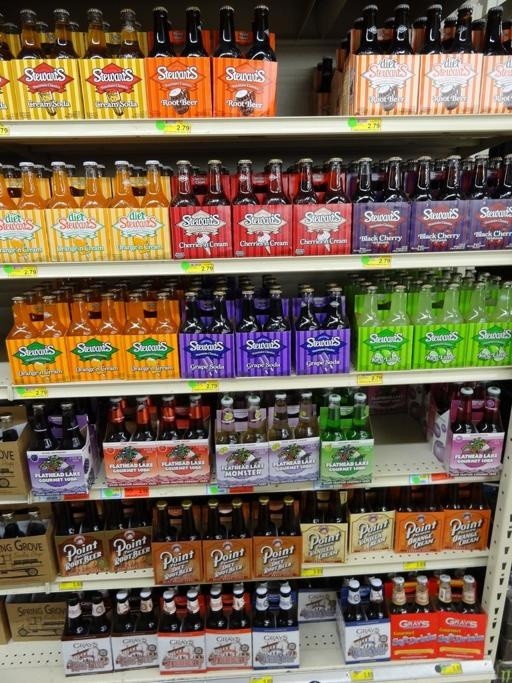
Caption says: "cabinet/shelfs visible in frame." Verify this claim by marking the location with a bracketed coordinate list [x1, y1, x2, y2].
[1, 0, 511, 683]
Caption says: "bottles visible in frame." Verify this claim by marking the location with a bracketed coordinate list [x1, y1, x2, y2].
[493, 282, 512, 322]
[416, 17, 429, 29]
[439, 156, 464, 202]
[299, 284, 311, 297]
[243, 395, 269, 442]
[346, 393, 374, 440]
[467, 155, 492, 200]
[349, 270, 507, 286]
[59, 403, 85, 450]
[381, 157, 409, 203]
[137, 588, 157, 634]
[303, 493, 320, 523]
[228, 500, 249, 538]
[482, 6, 506, 57]
[66, 295, 97, 336]
[461, 158, 475, 171]
[246, 6, 277, 59]
[6, 297, 38, 340]
[154, 502, 176, 538]
[202, 501, 226, 538]
[358, 286, 383, 327]
[152, 293, 178, 335]
[213, 8, 242, 58]
[413, 284, 436, 325]
[233, 160, 260, 204]
[322, 157, 348, 203]
[46, 162, 78, 208]
[2, 165, 16, 180]
[90, 593, 109, 637]
[357, 16, 369, 29]
[99, 294, 122, 335]
[206, 587, 228, 630]
[356, 6, 384, 55]
[221, 166, 229, 175]
[410, 156, 438, 202]
[22, 280, 179, 296]
[472, 19, 487, 30]
[127, 164, 136, 176]
[321, 393, 345, 441]
[185, 394, 210, 440]
[349, 488, 369, 514]
[421, 485, 437, 512]
[437, 284, 463, 324]
[105, 397, 131, 441]
[294, 392, 320, 440]
[132, 396, 156, 441]
[236, 290, 262, 332]
[158, 164, 165, 177]
[67, 593, 87, 638]
[458, 575, 480, 615]
[181, 590, 205, 633]
[264, 290, 289, 332]
[96, 165, 105, 178]
[133, 22, 146, 33]
[269, 393, 293, 440]
[465, 282, 492, 322]
[101, 22, 115, 33]
[445, 482, 462, 509]
[0, 413, 18, 443]
[229, 587, 250, 631]
[202, 160, 230, 206]
[317, 58, 335, 95]
[178, 501, 198, 538]
[29, 404, 58, 451]
[276, 584, 298, 627]
[388, 5, 415, 55]
[395, 488, 418, 512]
[351, 161, 360, 171]
[492, 156, 503, 171]
[81, 162, 107, 208]
[37, 296, 66, 337]
[17, 162, 45, 209]
[379, 160, 389, 169]
[326, 492, 344, 522]
[149, 7, 176, 58]
[17, 10, 48, 60]
[81, 9, 112, 60]
[34, 21, 49, 31]
[443, 18, 461, 28]
[192, 166, 201, 175]
[450, 4, 477, 54]
[263, 160, 289, 204]
[344, 578, 368, 622]
[326, 283, 337, 297]
[351, 157, 379, 203]
[3, 23, 22, 37]
[478, 387, 503, 433]
[470, 483, 486, 509]
[68, 22, 84, 33]
[252, 584, 273, 628]
[171, 161, 200, 206]
[408, 160, 418, 171]
[385, 18, 398, 30]
[109, 161, 139, 207]
[180, 292, 206, 334]
[295, 288, 321, 330]
[375, 489, 390, 512]
[157, 395, 183, 440]
[217, 396, 242, 444]
[34, 165, 47, 179]
[322, 288, 348, 329]
[254, 497, 276, 537]
[0, 14, 14, 61]
[497, 154, 512, 199]
[437, 575, 456, 613]
[388, 578, 410, 613]
[277, 496, 299, 536]
[179, 7, 208, 58]
[385, 286, 411, 326]
[419, 4, 446, 55]
[368, 577, 387, 621]
[186, 275, 285, 293]
[452, 387, 476, 434]
[2, 510, 24, 538]
[49, 9, 81, 57]
[25, 507, 47, 536]
[123, 294, 151, 335]
[412, 576, 434, 613]
[293, 158, 319, 204]
[0, 164, 18, 209]
[114, 591, 135, 633]
[158, 589, 182, 632]
[208, 292, 233, 334]
[116, 8, 145, 58]
[64, 165, 78, 178]
[141, 160, 170, 208]
[434, 159, 448, 172]
